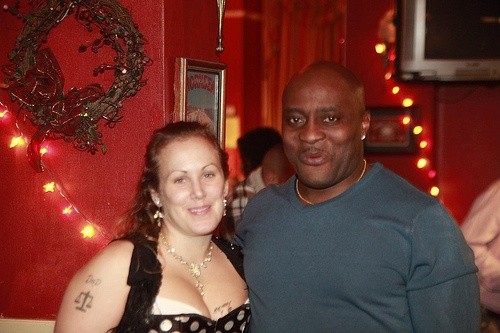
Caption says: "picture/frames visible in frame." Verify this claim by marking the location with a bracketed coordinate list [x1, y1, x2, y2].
[364, 105, 417, 155]
[173, 56, 227, 151]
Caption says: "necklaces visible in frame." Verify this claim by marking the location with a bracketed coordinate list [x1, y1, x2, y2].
[296, 159, 366, 205]
[158, 233, 213, 296]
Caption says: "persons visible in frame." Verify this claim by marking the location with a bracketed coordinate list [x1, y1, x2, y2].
[460, 179, 500, 333]
[231, 62, 480, 333]
[54, 121, 253, 333]
[221, 126, 293, 241]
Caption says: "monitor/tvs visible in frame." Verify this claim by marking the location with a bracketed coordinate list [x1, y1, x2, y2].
[394, 0, 500, 81]
